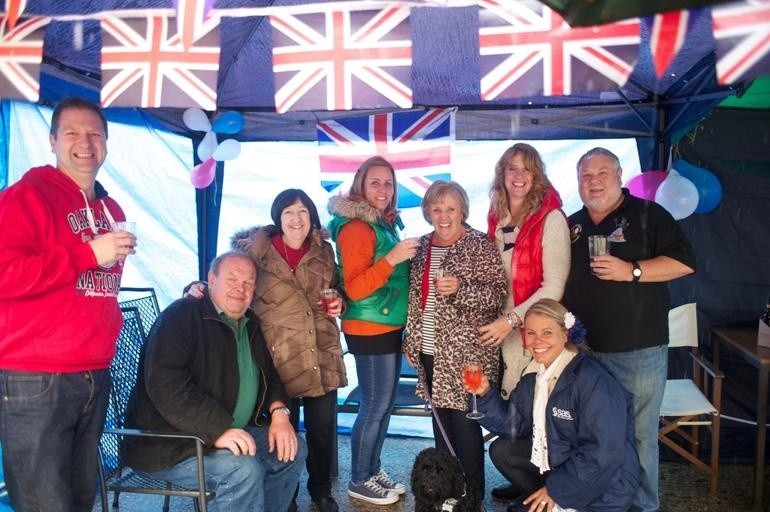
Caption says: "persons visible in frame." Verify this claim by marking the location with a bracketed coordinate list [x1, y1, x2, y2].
[460, 297, 643, 512]
[326, 155, 423, 507]
[485, 143, 572, 499]
[1, 96, 140, 511]
[558, 145, 698, 512]
[121, 250, 309, 512]
[400, 178, 509, 511]
[230, 187, 349, 512]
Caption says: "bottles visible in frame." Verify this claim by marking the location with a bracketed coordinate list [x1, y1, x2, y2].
[762, 304, 770, 326]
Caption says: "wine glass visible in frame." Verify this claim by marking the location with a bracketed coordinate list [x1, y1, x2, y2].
[461, 360, 484, 420]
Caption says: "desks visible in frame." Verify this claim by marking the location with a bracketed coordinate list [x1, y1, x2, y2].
[708, 323, 769, 506]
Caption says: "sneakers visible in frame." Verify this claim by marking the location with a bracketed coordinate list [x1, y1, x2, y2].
[372, 468, 406, 495]
[348, 476, 399, 505]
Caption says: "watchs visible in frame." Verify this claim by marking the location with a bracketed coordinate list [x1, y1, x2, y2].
[631, 261, 642, 283]
[269, 405, 292, 419]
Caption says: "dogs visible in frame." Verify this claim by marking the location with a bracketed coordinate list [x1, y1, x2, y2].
[409, 447, 481, 512]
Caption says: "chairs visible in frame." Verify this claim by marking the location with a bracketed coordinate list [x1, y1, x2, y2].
[656, 303, 722, 494]
[96, 307, 213, 512]
[111, 286, 160, 340]
[343, 330, 431, 414]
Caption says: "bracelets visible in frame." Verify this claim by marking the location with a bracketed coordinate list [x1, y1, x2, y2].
[506, 312, 517, 330]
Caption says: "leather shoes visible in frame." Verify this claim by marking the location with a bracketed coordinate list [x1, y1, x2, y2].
[491, 482, 543, 512]
[316, 493, 338, 512]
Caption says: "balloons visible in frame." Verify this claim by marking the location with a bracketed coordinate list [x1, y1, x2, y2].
[623, 158, 723, 222]
[182, 105, 246, 192]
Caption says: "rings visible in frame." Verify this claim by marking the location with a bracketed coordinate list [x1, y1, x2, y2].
[291, 442, 295, 445]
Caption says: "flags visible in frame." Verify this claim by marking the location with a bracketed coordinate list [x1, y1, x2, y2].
[478, 0, 642, 101]
[316, 107, 456, 215]
[1, 13, 51, 103]
[100, 10, 222, 111]
[271, 1, 412, 113]
[648, 1, 770, 96]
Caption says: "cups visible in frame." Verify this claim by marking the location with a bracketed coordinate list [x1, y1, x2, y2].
[588, 235, 613, 277]
[405, 233, 421, 255]
[432, 267, 447, 295]
[112, 221, 138, 252]
[319, 288, 338, 317]
